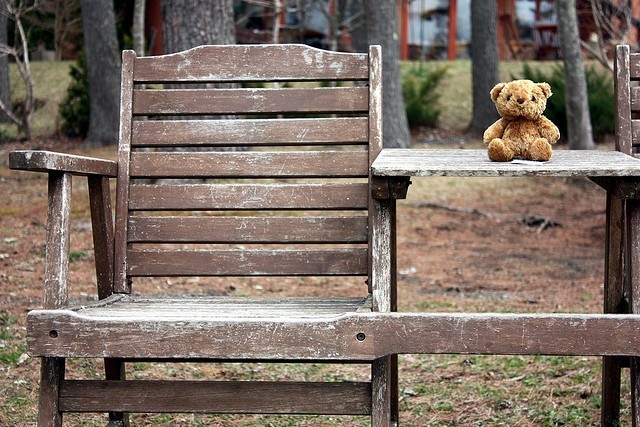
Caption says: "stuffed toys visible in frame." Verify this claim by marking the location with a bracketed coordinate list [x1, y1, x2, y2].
[482, 77, 561, 161]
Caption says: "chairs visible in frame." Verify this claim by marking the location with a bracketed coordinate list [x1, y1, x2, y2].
[7, 43, 411, 427]
[599, 44, 640, 427]
